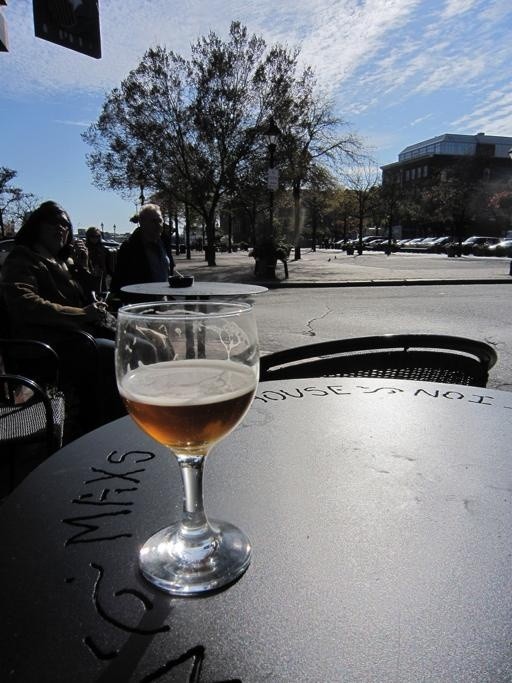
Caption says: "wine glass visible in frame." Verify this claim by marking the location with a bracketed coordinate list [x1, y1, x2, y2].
[114, 300, 260, 597]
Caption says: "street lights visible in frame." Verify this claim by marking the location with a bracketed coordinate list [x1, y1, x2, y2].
[113, 223, 116, 239]
[101, 222, 104, 237]
[259, 116, 282, 283]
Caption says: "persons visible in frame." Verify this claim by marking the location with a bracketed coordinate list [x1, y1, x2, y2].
[66, 201, 178, 368]
[0, 199, 138, 433]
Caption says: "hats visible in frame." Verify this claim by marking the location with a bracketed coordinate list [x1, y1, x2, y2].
[87, 229, 101, 236]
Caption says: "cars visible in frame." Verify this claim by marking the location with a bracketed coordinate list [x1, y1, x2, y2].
[333, 235, 512, 257]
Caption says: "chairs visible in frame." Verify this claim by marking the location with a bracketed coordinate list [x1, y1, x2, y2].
[259, 335, 497, 387]
[0, 337, 66, 453]
[0, 296, 117, 436]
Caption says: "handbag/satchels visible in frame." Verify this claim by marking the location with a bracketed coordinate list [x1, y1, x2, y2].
[79, 290, 117, 340]
